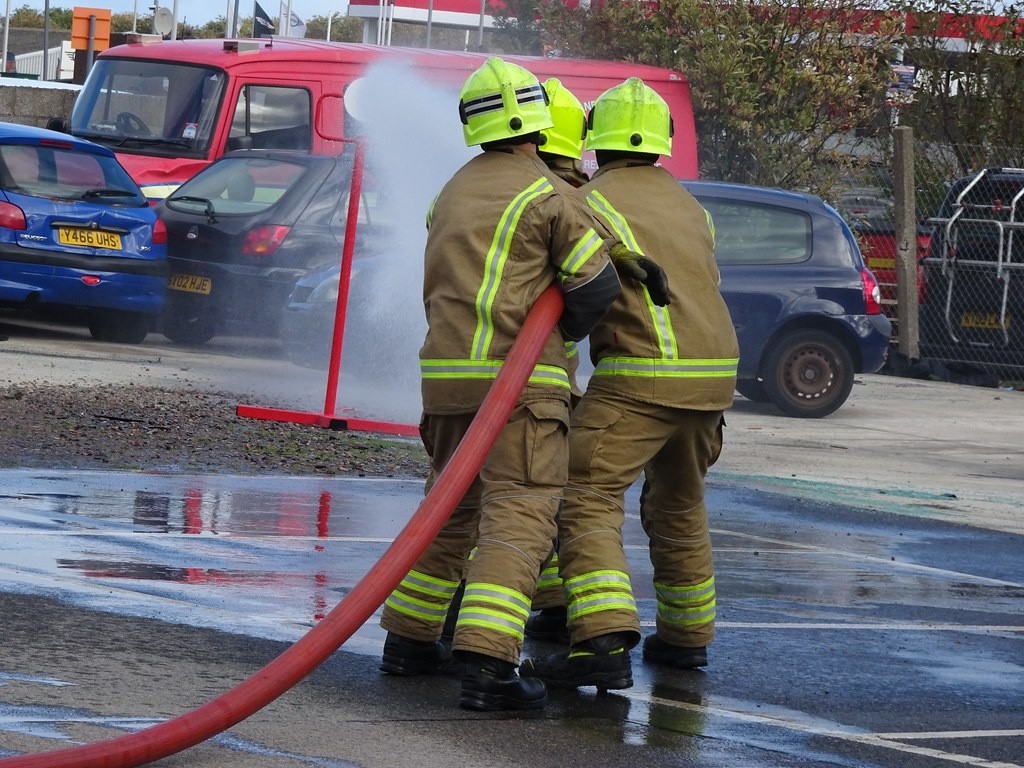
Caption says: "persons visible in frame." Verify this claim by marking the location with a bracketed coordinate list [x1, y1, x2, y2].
[376, 54, 743, 692]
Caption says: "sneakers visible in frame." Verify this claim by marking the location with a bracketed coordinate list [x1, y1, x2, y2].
[643, 635, 708, 668]
[378, 631, 445, 675]
[441, 581, 464, 638]
[459, 656, 547, 713]
[524, 607, 569, 642]
[518, 635, 633, 690]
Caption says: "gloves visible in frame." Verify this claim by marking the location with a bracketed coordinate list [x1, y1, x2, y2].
[609, 243, 671, 308]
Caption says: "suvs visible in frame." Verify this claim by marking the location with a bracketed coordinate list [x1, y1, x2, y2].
[918, 166, 1024, 380]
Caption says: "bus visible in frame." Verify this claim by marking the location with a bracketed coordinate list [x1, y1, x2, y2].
[46, 36, 699, 207]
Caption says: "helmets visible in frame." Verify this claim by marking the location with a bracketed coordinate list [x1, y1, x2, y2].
[539, 78, 589, 160]
[459, 55, 554, 147]
[585, 76, 674, 159]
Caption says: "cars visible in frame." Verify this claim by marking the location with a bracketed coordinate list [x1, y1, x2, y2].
[0, 123, 171, 344]
[151, 149, 426, 345]
[278, 179, 892, 419]
[825, 153, 933, 321]
